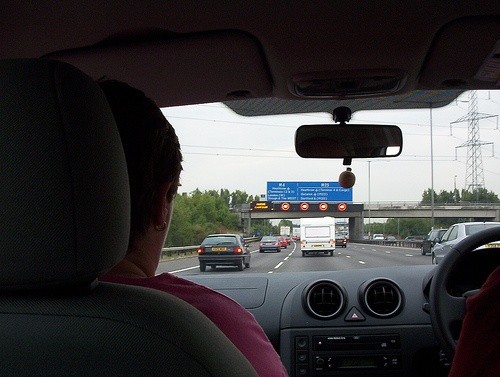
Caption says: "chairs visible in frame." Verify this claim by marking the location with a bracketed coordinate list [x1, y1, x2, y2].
[0, 57, 258, 377]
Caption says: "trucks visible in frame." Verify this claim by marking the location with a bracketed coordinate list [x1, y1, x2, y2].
[279, 225, 291, 237]
[299, 216, 336, 257]
[292, 227, 300, 241]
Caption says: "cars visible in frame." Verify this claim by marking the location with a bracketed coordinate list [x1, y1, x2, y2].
[384, 235, 397, 241]
[335, 233, 347, 248]
[258, 235, 282, 253]
[275, 235, 288, 249]
[283, 235, 292, 245]
[197, 233, 252, 272]
[402, 236, 425, 249]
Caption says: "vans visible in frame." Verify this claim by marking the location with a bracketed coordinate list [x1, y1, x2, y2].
[372, 233, 384, 240]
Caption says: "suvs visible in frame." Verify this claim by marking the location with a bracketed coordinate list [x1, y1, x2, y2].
[431, 219, 500, 265]
[421, 228, 449, 256]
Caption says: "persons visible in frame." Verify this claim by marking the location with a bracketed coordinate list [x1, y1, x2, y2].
[447, 267, 500, 377]
[95, 79, 290, 377]
[343, 127, 387, 155]
